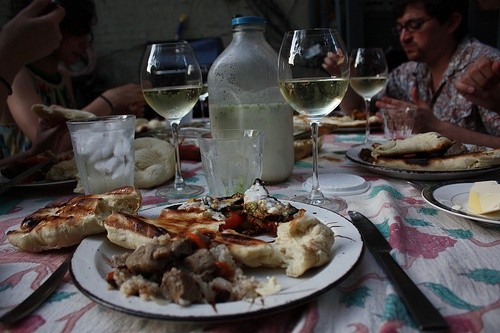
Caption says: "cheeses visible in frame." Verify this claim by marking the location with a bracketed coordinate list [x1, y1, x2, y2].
[469, 181, 500, 214]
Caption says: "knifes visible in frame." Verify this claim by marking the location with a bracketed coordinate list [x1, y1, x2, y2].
[346, 209, 452, 333]
[0, 255, 75, 326]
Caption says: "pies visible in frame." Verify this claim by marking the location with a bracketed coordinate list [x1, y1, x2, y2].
[291, 114, 500, 171]
[6, 105, 335, 277]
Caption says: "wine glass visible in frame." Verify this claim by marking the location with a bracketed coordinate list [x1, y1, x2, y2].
[346, 46, 389, 143]
[277, 26, 352, 209]
[140, 41, 204, 198]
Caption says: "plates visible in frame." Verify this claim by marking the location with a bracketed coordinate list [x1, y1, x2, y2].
[71, 198, 364, 321]
[0, 169, 77, 188]
[347, 140, 500, 180]
[290, 109, 386, 141]
[422, 179, 500, 227]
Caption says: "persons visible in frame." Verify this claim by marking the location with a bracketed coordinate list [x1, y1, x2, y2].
[454, 55, 500, 114]
[0, 0, 68, 170]
[0, 0, 146, 160]
[322, 0, 500, 147]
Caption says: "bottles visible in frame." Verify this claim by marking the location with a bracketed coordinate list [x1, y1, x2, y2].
[205, 15, 295, 184]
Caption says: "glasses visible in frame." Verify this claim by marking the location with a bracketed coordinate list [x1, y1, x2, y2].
[392, 16, 431, 34]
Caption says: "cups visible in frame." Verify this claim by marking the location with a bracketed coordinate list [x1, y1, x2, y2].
[67, 114, 135, 198]
[381, 102, 417, 138]
[196, 129, 263, 197]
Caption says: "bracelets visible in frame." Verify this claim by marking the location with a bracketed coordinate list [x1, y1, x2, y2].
[99, 94, 114, 113]
[0, 77, 12, 96]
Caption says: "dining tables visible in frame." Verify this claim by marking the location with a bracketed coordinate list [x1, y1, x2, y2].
[1, 127, 500, 333]
